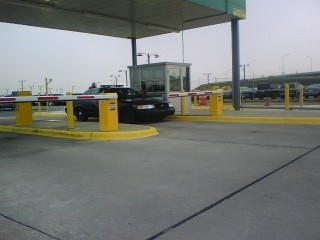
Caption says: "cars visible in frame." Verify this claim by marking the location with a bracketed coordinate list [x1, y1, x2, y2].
[63, 86, 175, 123]
[223, 91, 231, 98]
[303, 84, 320, 98]
[240, 82, 303, 98]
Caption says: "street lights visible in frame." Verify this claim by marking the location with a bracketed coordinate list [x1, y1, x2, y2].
[282, 54, 290, 72]
[137, 53, 160, 64]
[240, 63, 250, 86]
[202, 71, 213, 85]
[306, 55, 312, 72]
[110, 75, 120, 85]
[119, 68, 129, 86]
[5, 77, 63, 95]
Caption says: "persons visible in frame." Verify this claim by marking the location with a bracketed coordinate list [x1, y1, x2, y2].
[89, 82, 97, 89]
